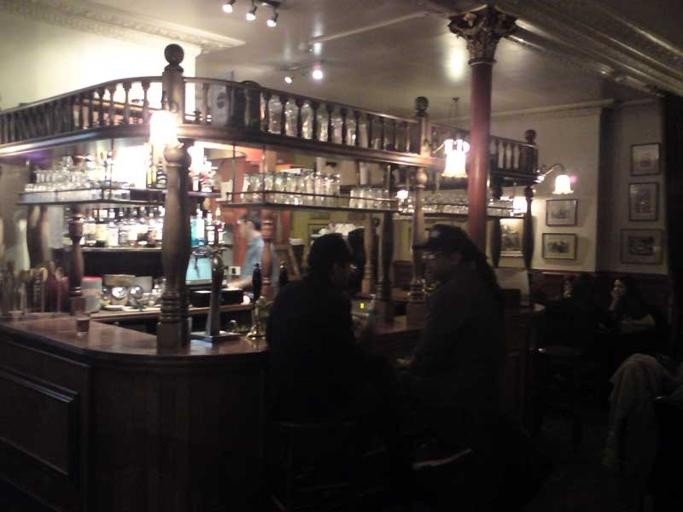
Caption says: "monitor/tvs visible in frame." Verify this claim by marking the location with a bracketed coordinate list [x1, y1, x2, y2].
[185, 252, 213, 284]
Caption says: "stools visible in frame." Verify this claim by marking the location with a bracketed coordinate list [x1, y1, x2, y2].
[415, 398, 501, 510]
[261, 419, 368, 510]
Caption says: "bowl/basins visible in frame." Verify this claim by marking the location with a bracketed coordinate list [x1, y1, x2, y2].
[103, 274, 152, 293]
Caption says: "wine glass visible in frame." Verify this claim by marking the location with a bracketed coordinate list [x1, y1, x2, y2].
[25, 166, 128, 200]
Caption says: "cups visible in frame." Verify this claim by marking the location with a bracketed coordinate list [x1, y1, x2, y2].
[241, 170, 391, 210]
[75, 316, 89, 336]
[402, 191, 513, 217]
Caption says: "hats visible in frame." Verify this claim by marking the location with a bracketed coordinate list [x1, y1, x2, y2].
[412, 223, 466, 254]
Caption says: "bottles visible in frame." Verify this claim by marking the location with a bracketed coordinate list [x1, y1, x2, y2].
[268, 93, 411, 152]
[0, 259, 101, 315]
[253, 261, 288, 302]
[63, 204, 224, 248]
[146, 144, 213, 193]
[489, 139, 527, 170]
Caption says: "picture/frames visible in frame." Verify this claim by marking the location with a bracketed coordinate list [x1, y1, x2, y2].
[545, 198, 578, 226]
[627, 181, 660, 222]
[619, 226, 664, 265]
[630, 144, 662, 177]
[540, 233, 577, 260]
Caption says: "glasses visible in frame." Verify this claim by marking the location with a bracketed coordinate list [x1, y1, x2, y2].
[417, 253, 441, 262]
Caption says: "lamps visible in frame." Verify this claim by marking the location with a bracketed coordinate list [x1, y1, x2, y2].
[533, 160, 575, 196]
[430, 96, 472, 181]
[278, 62, 326, 84]
[222, 0, 280, 28]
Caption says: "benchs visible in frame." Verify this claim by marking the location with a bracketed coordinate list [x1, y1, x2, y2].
[528, 267, 674, 329]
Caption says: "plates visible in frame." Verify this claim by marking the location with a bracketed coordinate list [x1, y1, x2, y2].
[104, 304, 162, 312]
